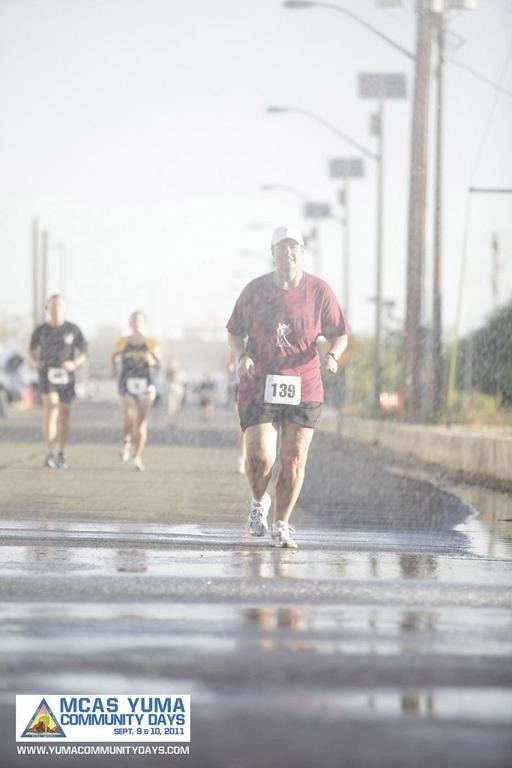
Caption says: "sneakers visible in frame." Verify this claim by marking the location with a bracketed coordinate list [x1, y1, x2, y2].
[45, 452, 67, 469]
[270, 519, 298, 549]
[247, 492, 271, 537]
[120, 446, 145, 470]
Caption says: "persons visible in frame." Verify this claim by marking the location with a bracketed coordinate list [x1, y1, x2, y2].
[110, 311, 161, 471]
[28, 295, 91, 470]
[225, 225, 354, 550]
[240, 549, 314, 648]
[0, 350, 239, 420]
[227, 354, 246, 475]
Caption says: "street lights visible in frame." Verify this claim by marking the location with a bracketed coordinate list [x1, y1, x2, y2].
[278, 1, 436, 417]
[249, 175, 354, 423]
[265, 98, 392, 418]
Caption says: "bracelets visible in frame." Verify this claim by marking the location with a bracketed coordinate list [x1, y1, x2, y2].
[236, 353, 247, 364]
[328, 352, 338, 362]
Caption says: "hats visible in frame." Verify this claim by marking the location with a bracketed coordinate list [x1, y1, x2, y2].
[271, 226, 304, 247]
